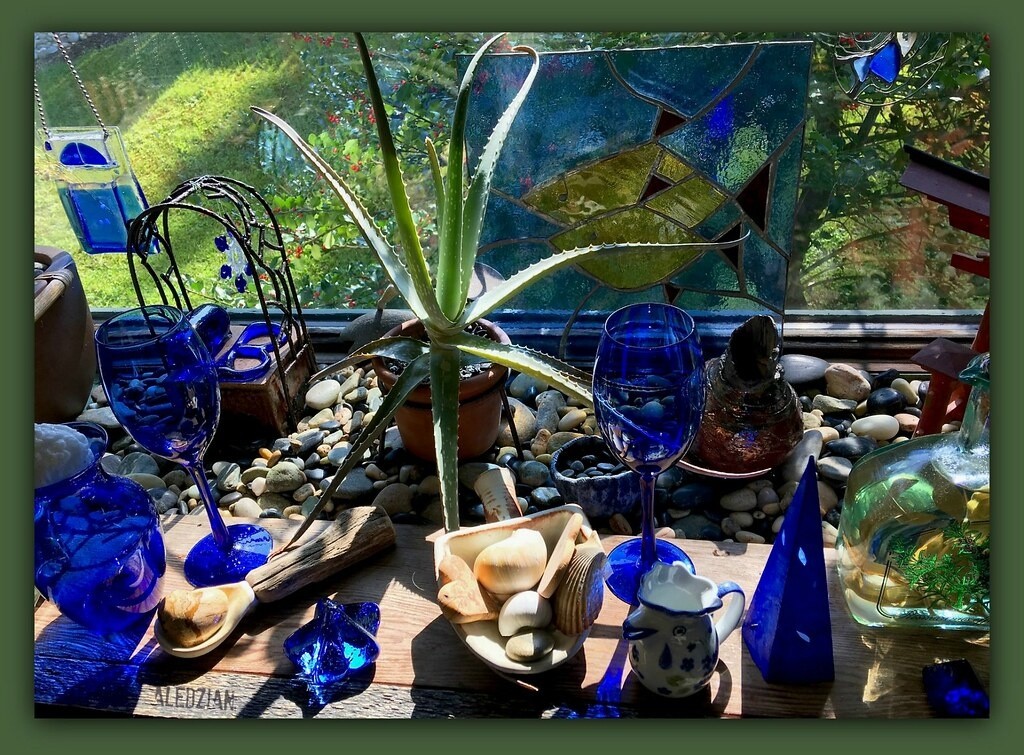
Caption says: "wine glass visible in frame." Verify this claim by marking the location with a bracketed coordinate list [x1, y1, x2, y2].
[95, 306, 273, 586]
[590, 304, 706, 605]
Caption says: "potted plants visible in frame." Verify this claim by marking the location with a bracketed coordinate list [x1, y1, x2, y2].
[248, 27, 594, 549]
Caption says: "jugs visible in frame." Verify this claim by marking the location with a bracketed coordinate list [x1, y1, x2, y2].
[622, 563, 745, 701]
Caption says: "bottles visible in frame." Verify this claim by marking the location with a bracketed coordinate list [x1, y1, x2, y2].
[34, 422, 168, 630]
[836, 355, 990, 630]
